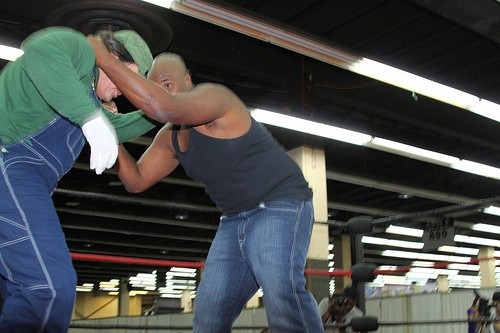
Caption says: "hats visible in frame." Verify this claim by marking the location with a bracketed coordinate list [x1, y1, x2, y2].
[114, 29, 153, 78]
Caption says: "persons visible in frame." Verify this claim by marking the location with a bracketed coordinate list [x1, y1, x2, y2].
[318, 287, 363, 333]
[86, 35, 324, 333]
[492, 292, 500, 333]
[467, 298, 482, 333]
[0, 27, 158, 333]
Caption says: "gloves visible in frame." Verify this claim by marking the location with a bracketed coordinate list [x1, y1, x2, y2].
[81, 113, 118, 174]
